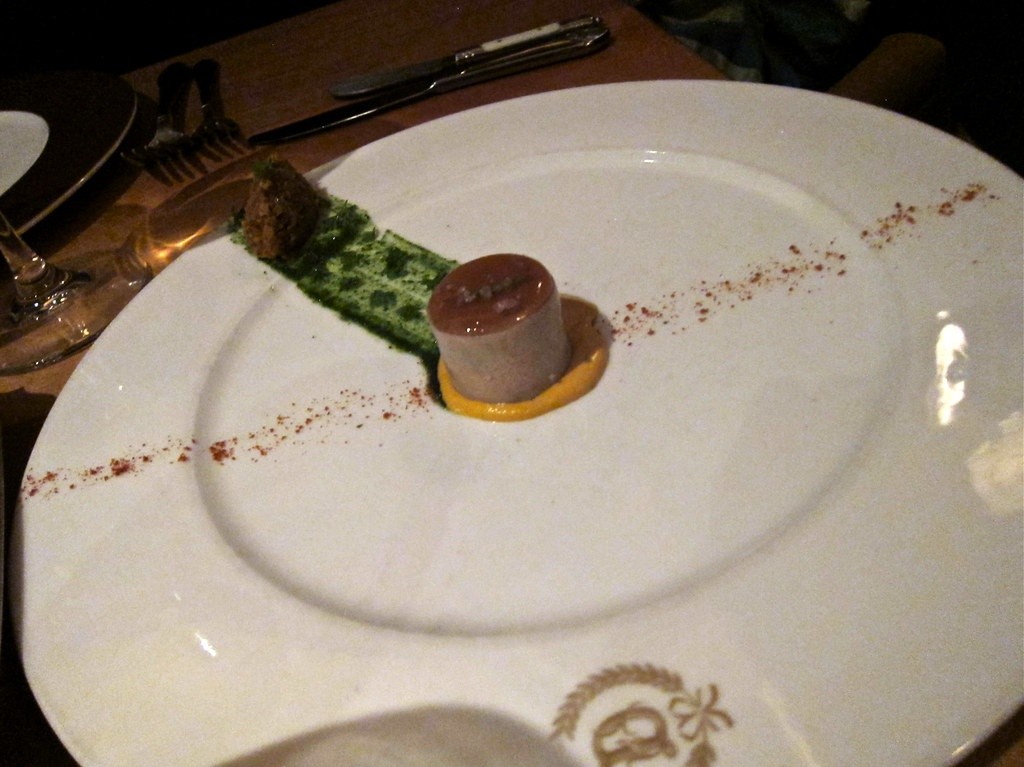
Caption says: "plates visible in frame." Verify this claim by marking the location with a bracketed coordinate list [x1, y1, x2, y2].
[9, 79, 1024, 767]
[0, 50, 137, 236]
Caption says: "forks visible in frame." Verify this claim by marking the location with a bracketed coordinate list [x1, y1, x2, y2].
[119, 59, 252, 186]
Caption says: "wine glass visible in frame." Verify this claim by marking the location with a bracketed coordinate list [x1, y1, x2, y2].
[0, 216, 152, 377]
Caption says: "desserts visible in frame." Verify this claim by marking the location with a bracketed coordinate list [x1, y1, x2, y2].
[425, 251, 610, 422]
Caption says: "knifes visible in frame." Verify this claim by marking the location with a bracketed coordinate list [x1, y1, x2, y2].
[248, 24, 610, 148]
[330, 14, 602, 95]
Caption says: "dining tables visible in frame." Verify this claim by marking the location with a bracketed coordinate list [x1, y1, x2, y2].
[0, 0, 1024, 767]
